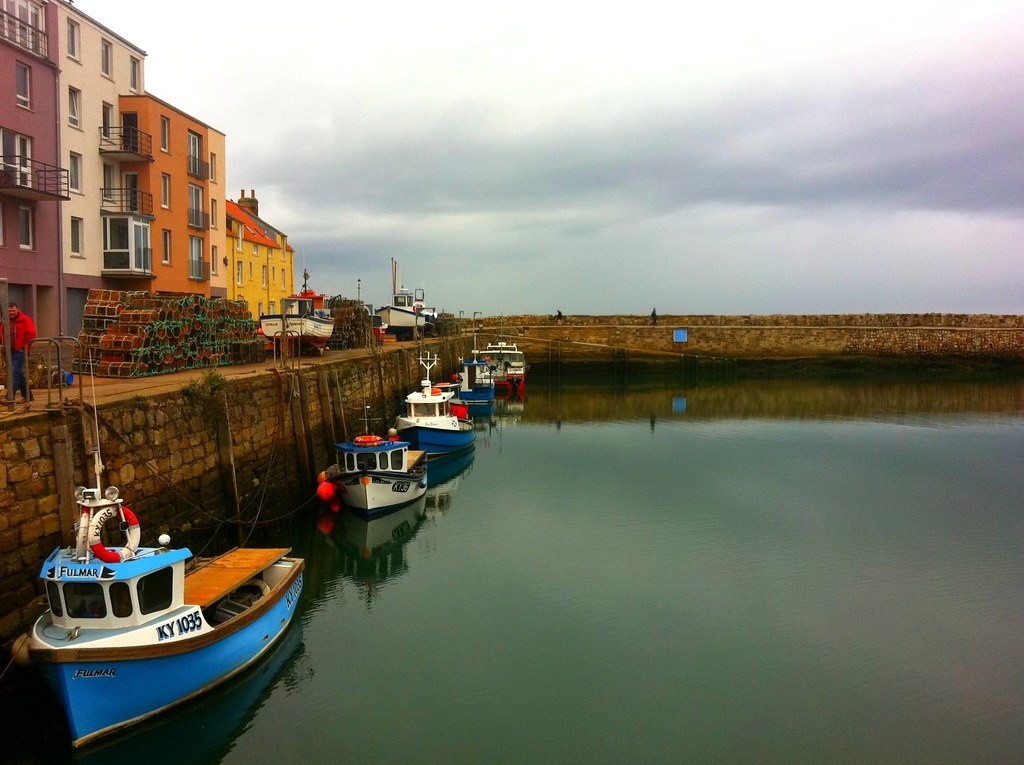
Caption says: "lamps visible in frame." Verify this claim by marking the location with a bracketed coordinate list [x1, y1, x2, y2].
[105, 485, 120, 501]
[73, 485, 88, 500]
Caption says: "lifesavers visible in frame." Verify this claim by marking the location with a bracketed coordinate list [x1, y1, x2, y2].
[437, 382, 450, 385]
[88, 505, 142, 563]
[413, 304, 423, 311]
[76, 505, 90, 561]
[354, 435, 383, 447]
[231, 577, 271, 606]
[422, 388, 442, 395]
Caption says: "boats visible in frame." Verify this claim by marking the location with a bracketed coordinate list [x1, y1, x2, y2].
[339, 492, 427, 582]
[29, 347, 307, 752]
[393, 351, 478, 464]
[258, 269, 337, 355]
[455, 312, 531, 408]
[422, 442, 477, 551]
[325, 392, 429, 516]
[373, 284, 428, 342]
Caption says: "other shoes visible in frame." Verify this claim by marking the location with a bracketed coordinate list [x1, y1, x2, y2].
[17, 395, 34, 404]
[0, 398, 7, 405]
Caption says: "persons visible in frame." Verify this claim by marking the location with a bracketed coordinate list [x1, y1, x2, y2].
[0, 301, 37, 406]
[557, 309, 563, 326]
[649, 308, 657, 326]
